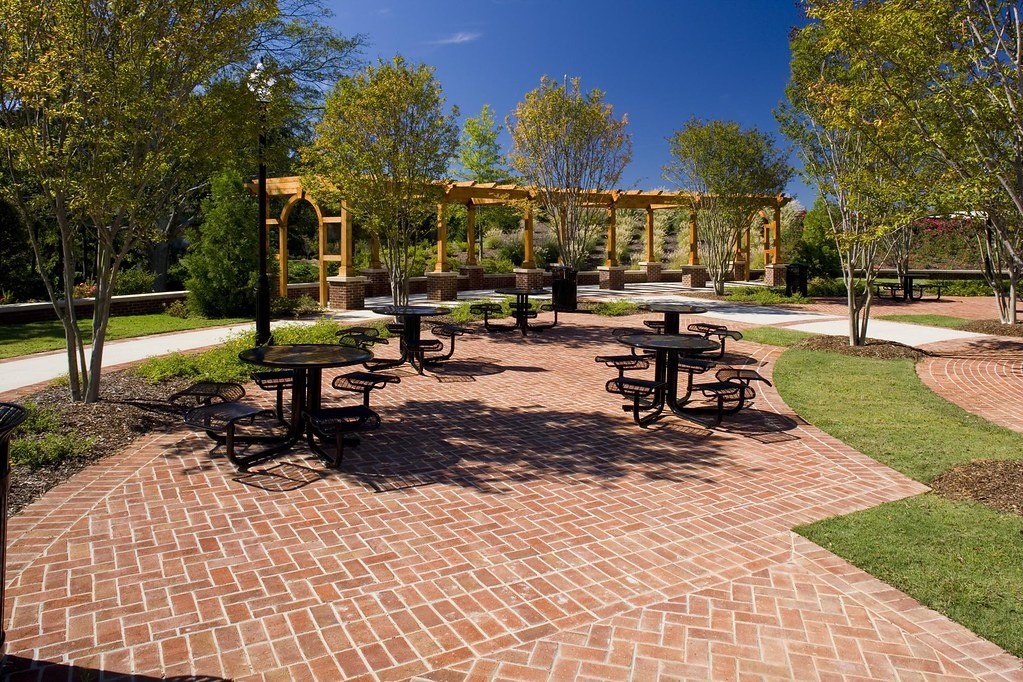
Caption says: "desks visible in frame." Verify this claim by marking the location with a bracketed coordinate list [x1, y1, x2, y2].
[888, 274, 929, 299]
[637, 304, 708, 335]
[494, 290, 550, 329]
[239, 343, 374, 454]
[371, 306, 451, 367]
[617, 334, 719, 423]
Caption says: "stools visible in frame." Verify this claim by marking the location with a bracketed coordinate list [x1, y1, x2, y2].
[643, 321, 664, 334]
[331, 372, 401, 424]
[168, 380, 245, 441]
[676, 358, 716, 396]
[339, 335, 388, 370]
[250, 371, 309, 420]
[469, 301, 562, 337]
[401, 339, 442, 374]
[611, 328, 654, 359]
[710, 330, 742, 361]
[311, 405, 380, 470]
[335, 326, 379, 362]
[687, 382, 755, 427]
[605, 377, 667, 426]
[594, 355, 649, 394]
[385, 324, 405, 355]
[184, 403, 275, 467]
[686, 323, 726, 340]
[431, 326, 474, 359]
[873, 283, 948, 300]
[714, 368, 772, 416]
